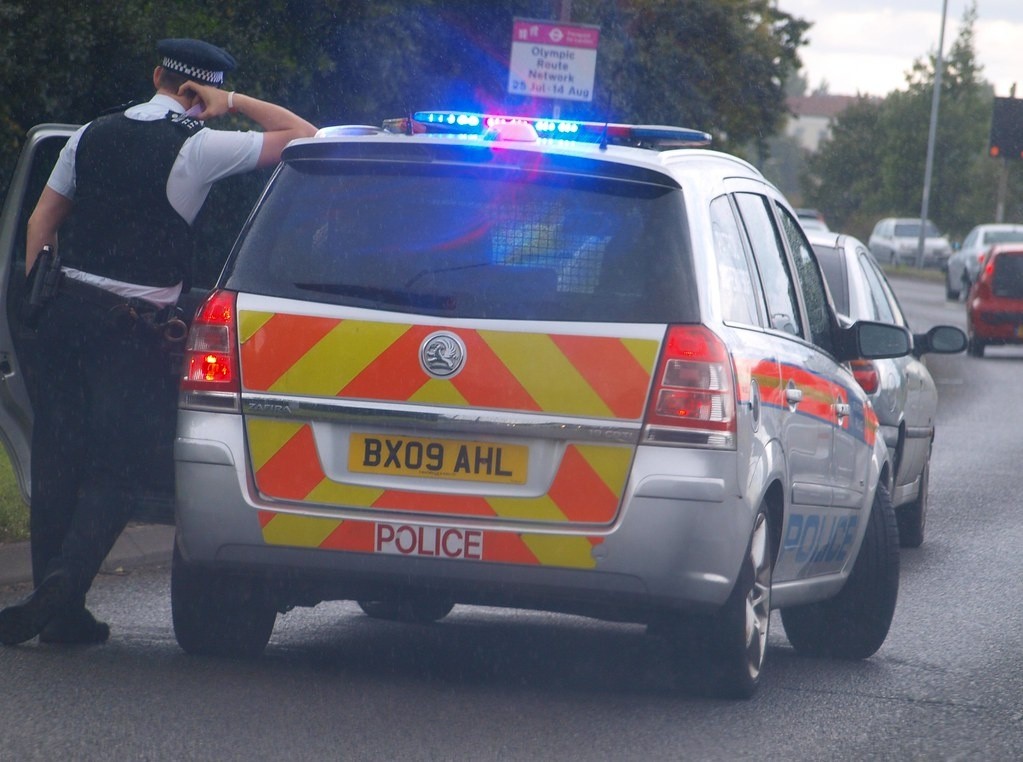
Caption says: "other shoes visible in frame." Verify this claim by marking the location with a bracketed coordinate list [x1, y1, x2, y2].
[0, 587, 60, 646]
[40, 614, 111, 646]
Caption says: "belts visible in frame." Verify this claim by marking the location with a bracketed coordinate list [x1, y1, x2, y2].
[60, 278, 170, 322]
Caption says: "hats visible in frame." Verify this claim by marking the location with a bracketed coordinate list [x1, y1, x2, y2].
[157, 38, 235, 87]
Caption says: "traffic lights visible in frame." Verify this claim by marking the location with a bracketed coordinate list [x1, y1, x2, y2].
[989, 96, 1023, 160]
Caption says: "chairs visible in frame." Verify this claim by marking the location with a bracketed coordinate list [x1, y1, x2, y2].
[593, 236, 646, 300]
[376, 201, 493, 263]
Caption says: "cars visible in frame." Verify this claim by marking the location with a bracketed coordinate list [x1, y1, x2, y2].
[867, 214, 956, 272]
[943, 222, 1022, 304]
[957, 242, 1022, 358]
[0, 110, 915, 699]
[791, 207, 972, 550]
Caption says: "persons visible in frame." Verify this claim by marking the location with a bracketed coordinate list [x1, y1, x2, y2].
[0, 37, 318, 653]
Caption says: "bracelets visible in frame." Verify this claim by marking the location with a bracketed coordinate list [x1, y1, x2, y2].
[227, 90, 235, 116]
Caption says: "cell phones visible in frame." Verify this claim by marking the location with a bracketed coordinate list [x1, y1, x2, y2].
[26, 246, 53, 327]
